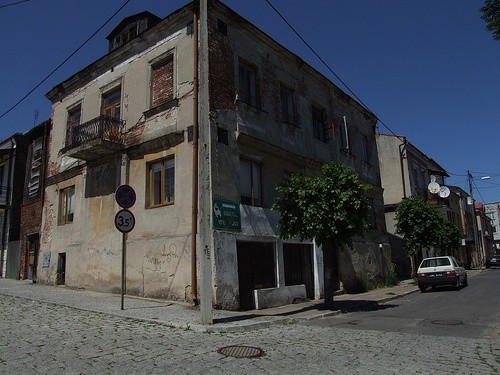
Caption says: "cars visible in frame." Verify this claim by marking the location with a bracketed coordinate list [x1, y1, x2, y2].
[486, 255, 500, 269]
[417, 255, 468, 292]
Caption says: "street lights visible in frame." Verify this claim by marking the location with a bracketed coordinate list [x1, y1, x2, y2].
[467, 170, 490, 270]
